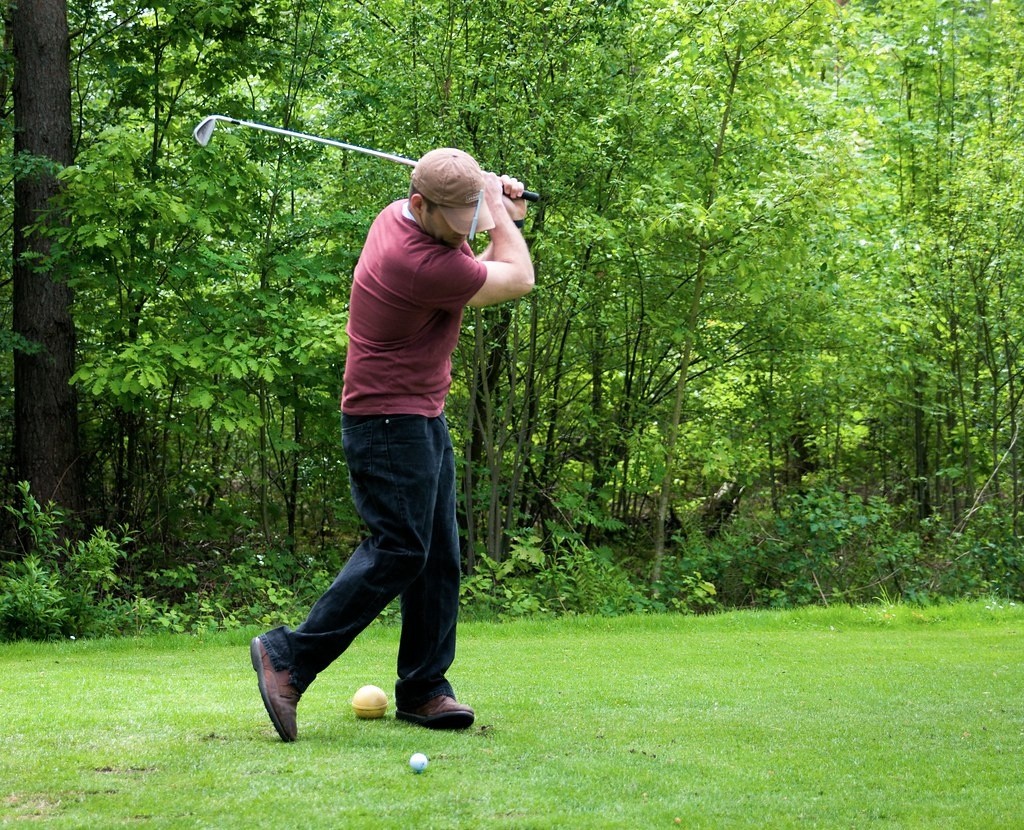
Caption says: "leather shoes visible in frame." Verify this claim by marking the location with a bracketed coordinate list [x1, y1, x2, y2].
[250, 636, 298, 742]
[396, 695, 474, 728]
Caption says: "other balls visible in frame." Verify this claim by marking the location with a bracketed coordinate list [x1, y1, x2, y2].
[352, 683, 389, 719]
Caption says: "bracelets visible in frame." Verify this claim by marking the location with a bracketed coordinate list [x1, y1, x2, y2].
[514, 220, 524, 228]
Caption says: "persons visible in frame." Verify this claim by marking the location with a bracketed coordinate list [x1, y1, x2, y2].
[250, 148, 534, 742]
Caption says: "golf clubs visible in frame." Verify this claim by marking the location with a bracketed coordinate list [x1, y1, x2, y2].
[195, 115, 541, 200]
[409, 753, 426, 768]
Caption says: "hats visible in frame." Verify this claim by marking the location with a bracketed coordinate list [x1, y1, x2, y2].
[411, 148, 495, 234]
